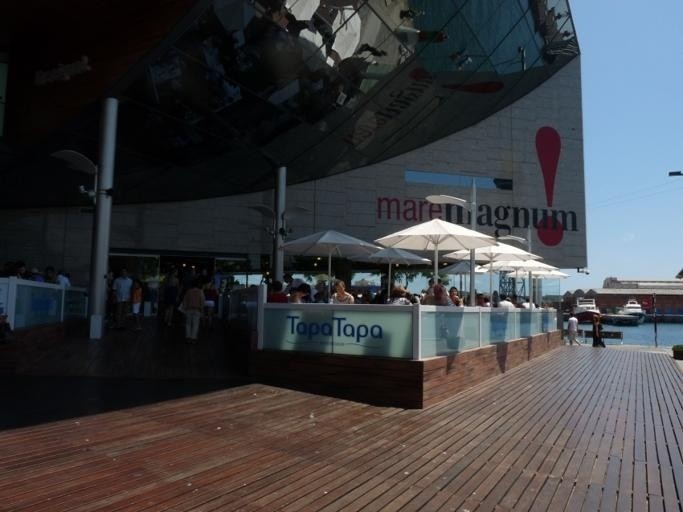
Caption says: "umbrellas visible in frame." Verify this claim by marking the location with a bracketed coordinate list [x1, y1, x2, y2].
[275, 215, 570, 310]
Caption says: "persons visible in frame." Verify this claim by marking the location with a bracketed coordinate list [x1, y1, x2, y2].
[592, 314, 606, 347]
[1, 260, 553, 345]
[566, 312, 581, 345]
[234, 1, 425, 132]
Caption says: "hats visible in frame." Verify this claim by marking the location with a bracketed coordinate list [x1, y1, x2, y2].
[314, 281, 327, 288]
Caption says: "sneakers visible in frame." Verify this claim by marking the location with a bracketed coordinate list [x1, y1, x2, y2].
[192, 339, 200, 345]
[186, 340, 191, 344]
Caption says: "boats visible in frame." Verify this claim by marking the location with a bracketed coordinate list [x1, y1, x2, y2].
[570, 297, 600, 324]
[623, 299, 646, 326]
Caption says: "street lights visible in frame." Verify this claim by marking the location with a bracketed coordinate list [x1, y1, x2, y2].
[59, 150, 99, 322]
[426, 177, 479, 308]
[498, 224, 534, 308]
[246, 204, 276, 285]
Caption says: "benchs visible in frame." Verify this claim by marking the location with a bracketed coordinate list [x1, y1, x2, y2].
[563, 327, 624, 344]
